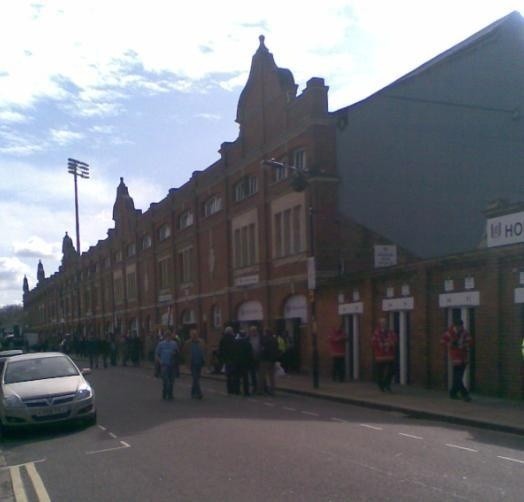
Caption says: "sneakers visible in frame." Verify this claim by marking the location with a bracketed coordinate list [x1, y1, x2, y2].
[449, 394, 472, 401]
[379, 383, 391, 391]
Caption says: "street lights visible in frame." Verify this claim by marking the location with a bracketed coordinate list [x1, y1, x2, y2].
[259, 157, 319, 388]
[68, 158, 89, 256]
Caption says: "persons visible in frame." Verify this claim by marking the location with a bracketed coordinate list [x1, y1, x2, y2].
[183, 328, 209, 401]
[0, 327, 143, 369]
[440, 319, 474, 403]
[219, 321, 287, 397]
[328, 325, 348, 383]
[154, 333, 179, 401]
[370, 317, 396, 394]
[161, 327, 182, 379]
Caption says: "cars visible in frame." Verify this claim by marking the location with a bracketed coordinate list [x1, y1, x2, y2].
[0, 350, 96, 429]
